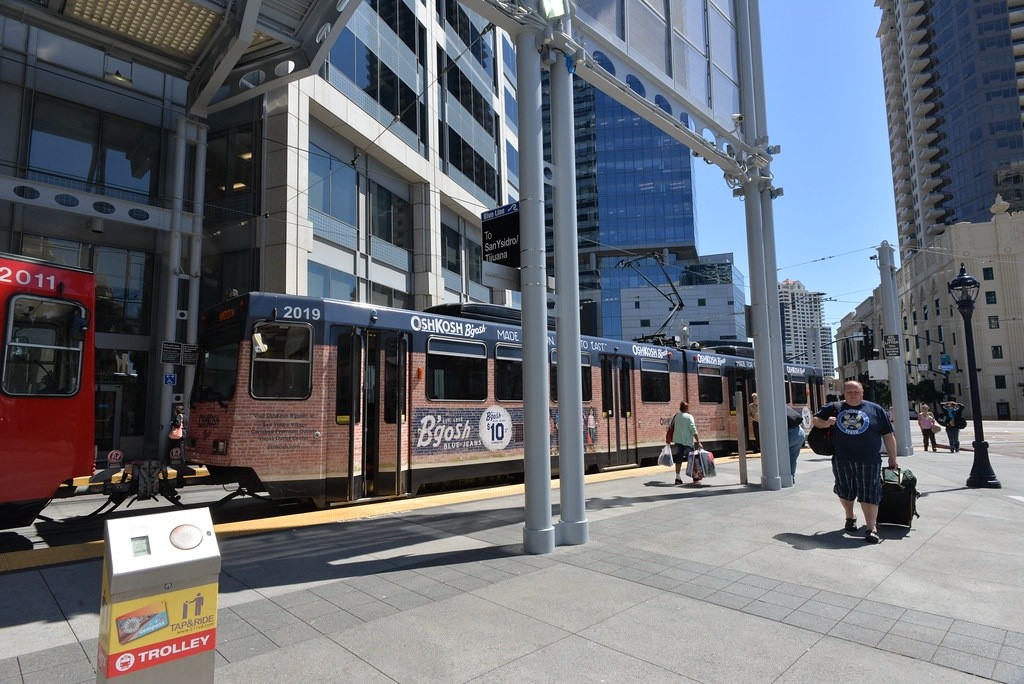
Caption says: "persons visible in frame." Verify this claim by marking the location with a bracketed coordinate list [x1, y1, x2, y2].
[940, 401, 964, 453]
[747, 393, 760, 451]
[787, 405, 805, 484]
[918, 404, 937, 452]
[889, 407, 894, 424]
[669, 401, 703, 485]
[813, 380, 898, 543]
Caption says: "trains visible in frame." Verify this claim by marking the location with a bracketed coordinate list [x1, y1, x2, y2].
[0, 248, 829, 531]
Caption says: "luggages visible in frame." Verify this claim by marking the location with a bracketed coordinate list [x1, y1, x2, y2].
[875, 466, 921, 528]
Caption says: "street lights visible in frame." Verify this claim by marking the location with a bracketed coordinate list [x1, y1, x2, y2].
[946, 263, 1002, 488]
[779, 292, 828, 363]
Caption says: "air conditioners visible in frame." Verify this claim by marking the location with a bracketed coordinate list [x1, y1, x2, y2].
[176, 310, 189, 319]
[171, 394, 184, 403]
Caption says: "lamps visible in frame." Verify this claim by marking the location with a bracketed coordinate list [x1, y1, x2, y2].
[88, 219, 105, 235]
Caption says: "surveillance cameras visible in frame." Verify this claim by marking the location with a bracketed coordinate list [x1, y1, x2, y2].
[731, 114, 745, 122]
[870, 254, 877, 260]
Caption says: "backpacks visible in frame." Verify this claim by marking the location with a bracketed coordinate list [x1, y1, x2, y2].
[786, 404, 803, 427]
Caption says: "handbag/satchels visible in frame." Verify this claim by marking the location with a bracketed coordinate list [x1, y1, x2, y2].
[685, 447, 716, 478]
[955, 409, 966, 429]
[666, 413, 676, 443]
[657, 444, 674, 467]
[807, 402, 840, 456]
[937, 408, 951, 425]
[169, 413, 183, 439]
[931, 420, 941, 433]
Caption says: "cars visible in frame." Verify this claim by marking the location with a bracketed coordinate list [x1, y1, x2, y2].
[909, 408, 918, 419]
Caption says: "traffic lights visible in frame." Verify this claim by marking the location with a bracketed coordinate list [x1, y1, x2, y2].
[863, 325, 874, 349]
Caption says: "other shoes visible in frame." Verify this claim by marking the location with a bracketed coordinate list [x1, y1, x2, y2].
[925, 447, 927, 451]
[865, 524, 879, 543]
[933, 448, 936, 451]
[951, 447, 958, 452]
[693, 477, 702, 483]
[845, 515, 857, 531]
[675, 479, 682, 485]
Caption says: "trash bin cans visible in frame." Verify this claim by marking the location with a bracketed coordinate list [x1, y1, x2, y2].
[96, 507, 221, 684]
